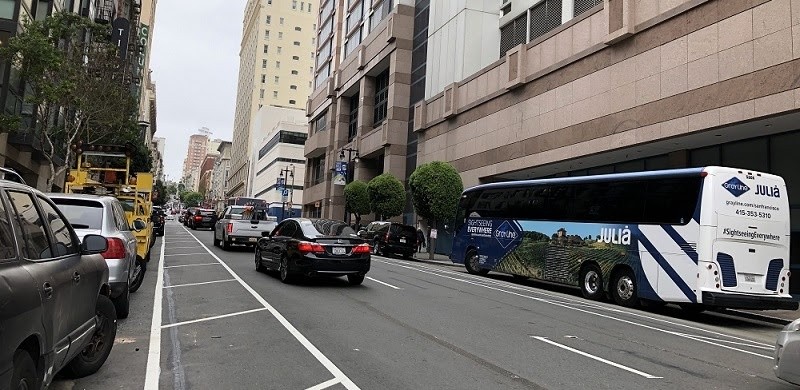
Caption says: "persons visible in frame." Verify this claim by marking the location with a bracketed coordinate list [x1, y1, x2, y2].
[417, 228, 426, 253]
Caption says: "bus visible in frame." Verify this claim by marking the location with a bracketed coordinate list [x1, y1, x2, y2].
[228, 197, 270, 212]
[449, 165, 799, 315]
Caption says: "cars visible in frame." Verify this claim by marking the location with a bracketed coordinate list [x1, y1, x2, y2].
[0, 170, 119, 390]
[773, 318, 800, 386]
[179, 206, 218, 231]
[42, 191, 165, 318]
[254, 217, 371, 286]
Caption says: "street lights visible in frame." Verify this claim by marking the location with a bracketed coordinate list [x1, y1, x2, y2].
[339, 147, 361, 227]
[280, 166, 294, 219]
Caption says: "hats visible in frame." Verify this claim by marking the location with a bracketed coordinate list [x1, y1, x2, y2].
[105, 161, 111, 165]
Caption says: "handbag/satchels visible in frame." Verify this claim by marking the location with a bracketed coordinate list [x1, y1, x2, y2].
[423, 242, 426, 248]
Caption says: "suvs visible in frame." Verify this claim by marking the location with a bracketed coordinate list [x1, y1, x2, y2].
[360, 220, 418, 258]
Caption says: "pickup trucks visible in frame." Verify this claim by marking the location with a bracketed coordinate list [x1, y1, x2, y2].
[212, 204, 280, 251]
